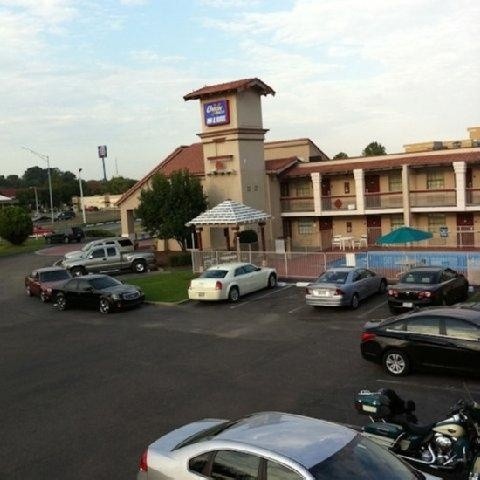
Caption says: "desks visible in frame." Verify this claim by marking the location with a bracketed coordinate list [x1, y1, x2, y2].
[340, 237, 355, 251]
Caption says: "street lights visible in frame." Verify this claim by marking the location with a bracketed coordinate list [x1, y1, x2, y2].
[22, 145, 87, 226]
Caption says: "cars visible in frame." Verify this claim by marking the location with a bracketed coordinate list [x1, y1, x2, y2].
[28, 204, 100, 245]
[24, 265, 74, 303]
[50, 272, 147, 315]
[135, 409, 446, 480]
[304, 266, 388, 312]
[383, 264, 470, 314]
[188, 260, 279, 306]
[359, 303, 479, 383]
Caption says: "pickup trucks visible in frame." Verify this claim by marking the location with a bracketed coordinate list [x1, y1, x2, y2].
[60, 243, 160, 277]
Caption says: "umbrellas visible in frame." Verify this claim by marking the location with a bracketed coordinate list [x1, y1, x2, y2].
[377, 227, 433, 249]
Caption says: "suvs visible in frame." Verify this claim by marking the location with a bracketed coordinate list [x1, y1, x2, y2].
[63, 235, 135, 261]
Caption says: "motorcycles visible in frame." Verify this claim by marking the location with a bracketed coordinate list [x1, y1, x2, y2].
[351, 385, 480, 480]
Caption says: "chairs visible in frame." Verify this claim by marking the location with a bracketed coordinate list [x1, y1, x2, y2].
[331, 235, 342, 250]
[358, 235, 367, 249]
